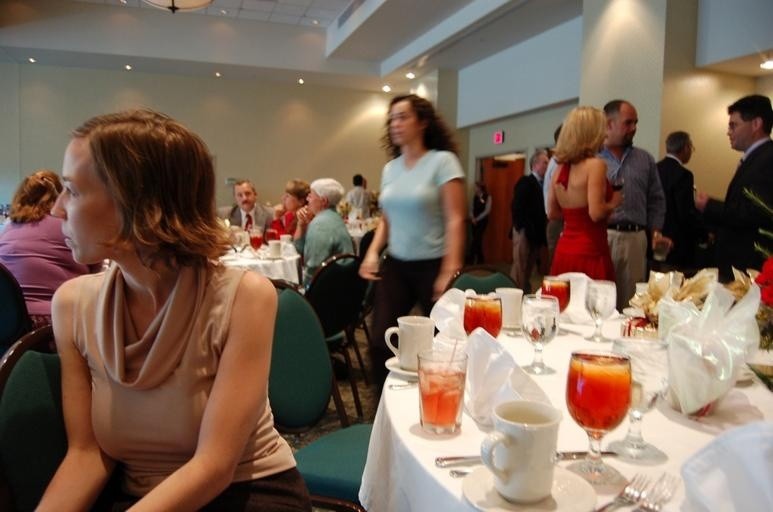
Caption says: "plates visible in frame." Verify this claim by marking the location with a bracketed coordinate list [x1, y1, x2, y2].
[621, 304, 648, 320]
[383, 354, 417, 381]
[460, 465, 597, 511]
[747, 349, 772, 377]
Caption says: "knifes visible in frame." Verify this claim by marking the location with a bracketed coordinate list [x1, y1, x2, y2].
[434, 450, 617, 467]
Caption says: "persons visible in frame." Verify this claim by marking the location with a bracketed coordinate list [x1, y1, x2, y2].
[357, 93, 470, 392]
[29, 106, 313, 511]
[506, 153, 551, 293]
[695, 94, 773, 285]
[464, 183, 494, 266]
[220, 178, 274, 228]
[343, 174, 375, 222]
[0, 171, 101, 354]
[268, 179, 312, 265]
[546, 105, 624, 289]
[542, 124, 567, 256]
[289, 178, 354, 288]
[593, 99, 672, 314]
[653, 131, 714, 277]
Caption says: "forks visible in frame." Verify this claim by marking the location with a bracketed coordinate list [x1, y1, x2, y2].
[592, 471, 683, 511]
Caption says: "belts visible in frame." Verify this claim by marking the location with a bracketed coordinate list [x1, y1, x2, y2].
[607, 224, 644, 231]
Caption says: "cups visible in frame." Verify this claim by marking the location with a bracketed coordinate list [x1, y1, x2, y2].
[481, 400, 561, 505]
[268, 240, 283, 256]
[383, 286, 564, 501]
[542, 277, 570, 314]
[417, 349, 469, 440]
[279, 235, 291, 251]
[384, 314, 435, 371]
[463, 294, 503, 338]
[496, 287, 523, 329]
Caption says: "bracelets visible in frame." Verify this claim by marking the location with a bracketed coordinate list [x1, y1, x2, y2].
[651, 232, 663, 237]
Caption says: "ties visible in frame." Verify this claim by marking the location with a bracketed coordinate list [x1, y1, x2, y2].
[244, 214, 252, 233]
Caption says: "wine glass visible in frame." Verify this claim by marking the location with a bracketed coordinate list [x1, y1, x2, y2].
[567, 351, 633, 482]
[521, 293, 560, 375]
[232, 228, 277, 260]
[612, 336, 673, 464]
[584, 279, 617, 342]
